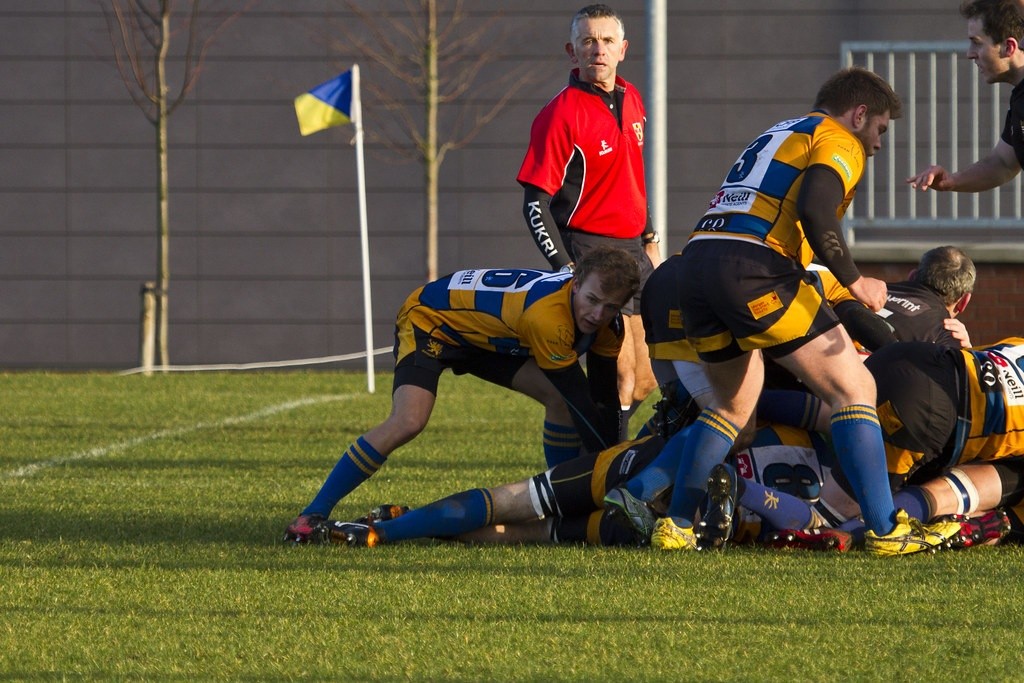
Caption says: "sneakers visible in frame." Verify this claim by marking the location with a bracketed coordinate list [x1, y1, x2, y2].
[308, 519, 386, 547]
[864, 507, 962, 556]
[352, 504, 409, 523]
[602, 483, 659, 537]
[651, 516, 696, 550]
[926, 510, 1011, 554]
[696, 462, 738, 551]
[282, 513, 324, 542]
[773, 526, 852, 552]
[652, 379, 701, 444]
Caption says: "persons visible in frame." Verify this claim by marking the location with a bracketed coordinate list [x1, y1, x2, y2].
[516, 4, 659, 446]
[282, 246, 641, 546]
[651, 67, 963, 557]
[906, 0, 1024, 192]
[298, 246, 1024, 550]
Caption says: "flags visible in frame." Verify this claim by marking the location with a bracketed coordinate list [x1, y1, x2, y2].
[294, 70, 356, 136]
